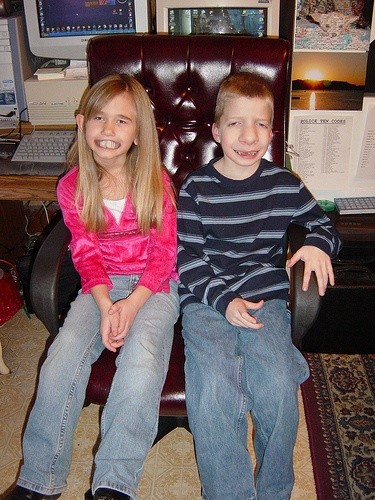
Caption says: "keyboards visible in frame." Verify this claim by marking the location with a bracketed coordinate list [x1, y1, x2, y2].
[11, 136, 76, 162]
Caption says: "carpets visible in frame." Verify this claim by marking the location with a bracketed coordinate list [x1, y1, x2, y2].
[300, 352, 375, 500]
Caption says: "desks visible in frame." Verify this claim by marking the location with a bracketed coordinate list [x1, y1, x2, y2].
[0, 121, 76, 200]
[28, 33, 321, 444]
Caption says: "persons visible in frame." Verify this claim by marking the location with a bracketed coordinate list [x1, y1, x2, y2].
[176, 73, 336, 500]
[0, 75, 178, 500]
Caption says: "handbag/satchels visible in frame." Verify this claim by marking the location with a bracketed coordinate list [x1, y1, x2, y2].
[0, 259, 24, 326]
[17, 234, 39, 314]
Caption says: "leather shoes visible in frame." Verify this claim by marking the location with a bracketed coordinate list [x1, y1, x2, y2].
[6, 485, 61, 500]
[94, 487, 130, 500]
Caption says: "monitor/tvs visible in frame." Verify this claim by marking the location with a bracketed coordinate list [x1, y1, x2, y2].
[24, 0, 149, 76]
[156, 0, 280, 37]
